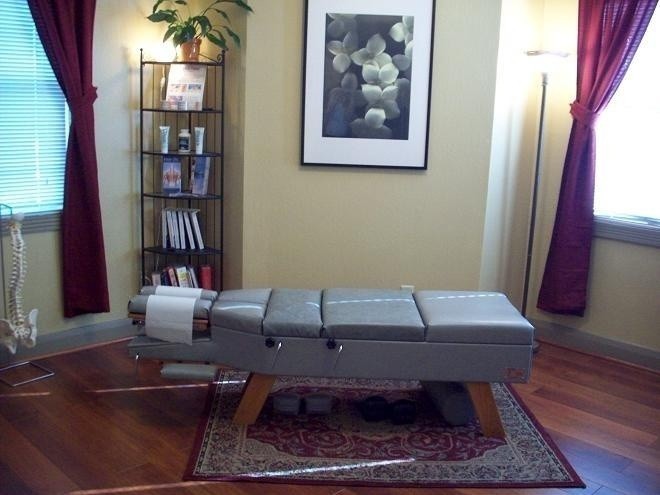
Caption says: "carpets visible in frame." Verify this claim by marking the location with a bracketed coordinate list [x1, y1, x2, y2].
[180, 367, 587, 488]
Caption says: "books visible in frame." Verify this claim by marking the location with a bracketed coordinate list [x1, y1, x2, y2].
[165, 64, 208, 112]
[150, 208, 212, 290]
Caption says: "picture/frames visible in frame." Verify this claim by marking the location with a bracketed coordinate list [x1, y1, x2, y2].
[301, 0, 437, 170]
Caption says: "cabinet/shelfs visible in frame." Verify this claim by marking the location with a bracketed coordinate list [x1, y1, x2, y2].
[140, 46, 225, 292]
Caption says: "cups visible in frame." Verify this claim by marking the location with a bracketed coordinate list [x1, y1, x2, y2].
[199, 264, 212, 290]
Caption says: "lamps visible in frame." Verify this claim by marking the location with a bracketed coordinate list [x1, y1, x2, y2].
[518, 44, 570, 314]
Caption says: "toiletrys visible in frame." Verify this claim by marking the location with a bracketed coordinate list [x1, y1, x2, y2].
[162, 265, 198, 287]
[151, 273, 160, 287]
[201, 264, 212, 288]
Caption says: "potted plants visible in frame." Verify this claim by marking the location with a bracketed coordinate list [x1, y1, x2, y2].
[145, 0, 254, 63]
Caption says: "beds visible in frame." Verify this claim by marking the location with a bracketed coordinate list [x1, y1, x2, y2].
[125, 284, 536, 439]
